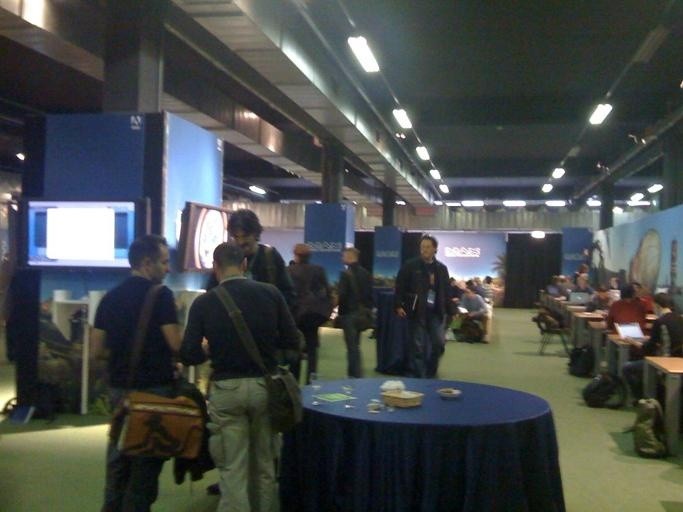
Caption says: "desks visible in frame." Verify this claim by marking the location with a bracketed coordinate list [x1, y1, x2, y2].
[531, 288, 682, 458]
[298, 378, 565, 510]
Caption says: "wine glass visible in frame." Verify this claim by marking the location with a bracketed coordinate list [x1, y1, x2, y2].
[307, 372, 326, 406]
[339, 374, 355, 408]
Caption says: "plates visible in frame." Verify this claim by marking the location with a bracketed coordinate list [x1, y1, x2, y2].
[438, 389, 461, 398]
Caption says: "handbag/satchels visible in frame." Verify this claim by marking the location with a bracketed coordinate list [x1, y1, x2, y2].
[108, 391, 203, 459]
[266, 373, 303, 433]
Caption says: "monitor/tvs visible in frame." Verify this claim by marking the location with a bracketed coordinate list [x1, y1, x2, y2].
[18, 195, 151, 275]
[179, 201, 236, 271]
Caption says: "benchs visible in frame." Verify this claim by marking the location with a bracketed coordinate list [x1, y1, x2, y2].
[484, 296, 494, 342]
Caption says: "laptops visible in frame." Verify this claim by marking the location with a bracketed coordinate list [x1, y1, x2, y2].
[614, 321, 645, 342]
[570, 292, 589, 304]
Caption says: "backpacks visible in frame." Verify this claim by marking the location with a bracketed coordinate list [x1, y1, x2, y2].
[623, 398, 667, 458]
[582, 371, 627, 410]
[568, 344, 596, 378]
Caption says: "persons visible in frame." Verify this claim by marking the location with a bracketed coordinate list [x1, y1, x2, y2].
[283, 233, 497, 381]
[544, 252, 682, 407]
[204, 208, 299, 381]
[91, 231, 211, 510]
[178, 243, 306, 510]
